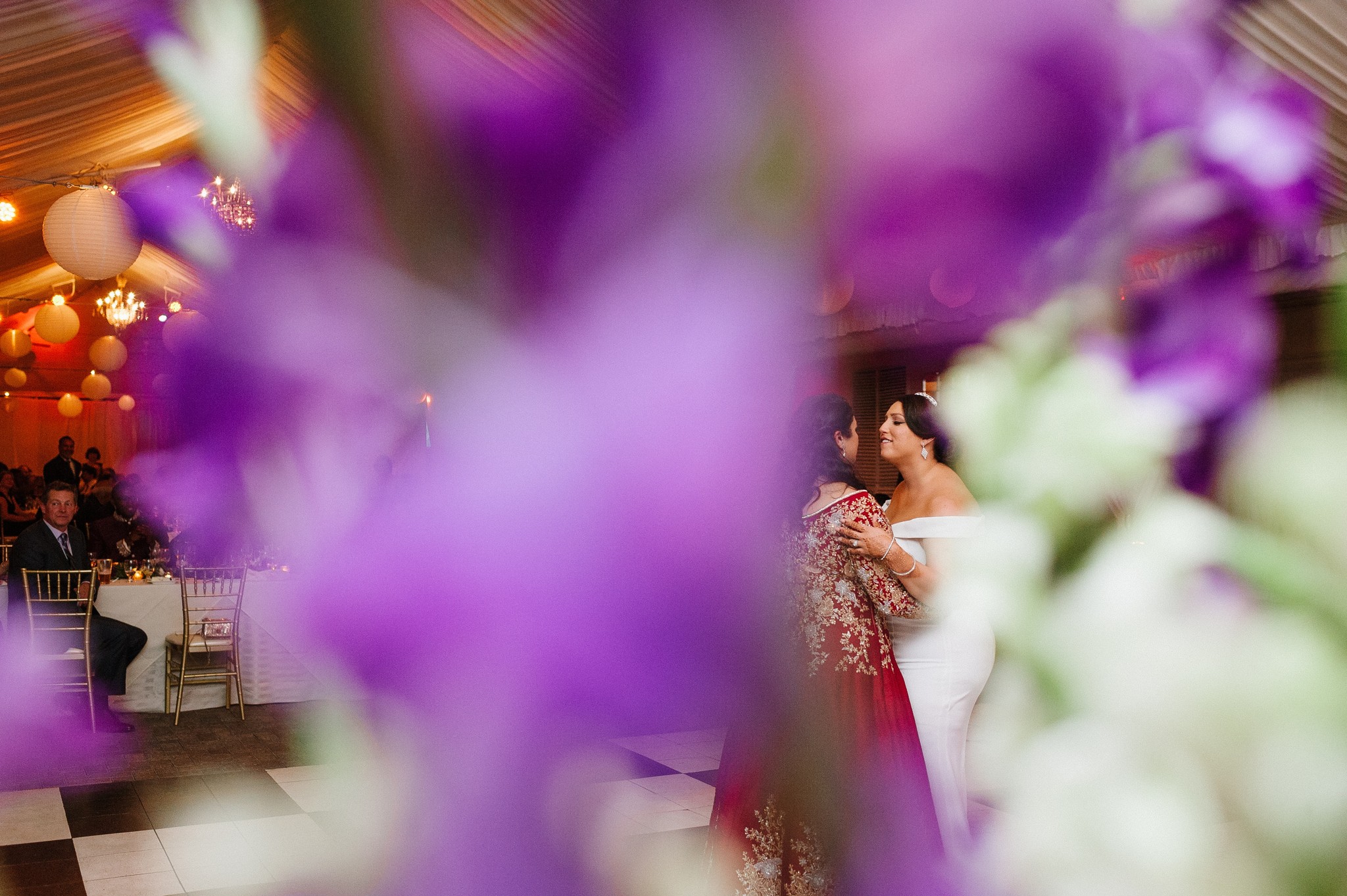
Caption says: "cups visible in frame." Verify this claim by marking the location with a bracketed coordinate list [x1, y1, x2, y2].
[150, 548, 171, 566]
[96, 559, 112, 586]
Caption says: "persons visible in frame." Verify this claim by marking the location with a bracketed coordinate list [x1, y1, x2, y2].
[698, 392, 953, 896]
[836, 390, 1003, 878]
[8, 481, 148, 733]
[0, 427, 268, 578]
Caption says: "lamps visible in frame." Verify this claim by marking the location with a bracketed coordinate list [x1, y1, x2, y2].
[0, 170, 260, 418]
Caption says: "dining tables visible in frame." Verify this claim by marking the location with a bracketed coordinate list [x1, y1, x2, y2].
[0, 568, 334, 716]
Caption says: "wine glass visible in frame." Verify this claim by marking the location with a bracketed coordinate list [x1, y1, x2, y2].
[142, 559, 155, 583]
[174, 537, 279, 580]
[123, 558, 139, 584]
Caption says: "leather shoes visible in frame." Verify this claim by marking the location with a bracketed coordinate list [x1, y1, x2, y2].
[83, 708, 136, 733]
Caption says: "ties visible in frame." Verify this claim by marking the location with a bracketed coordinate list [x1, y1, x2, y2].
[60, 532, 73, 561]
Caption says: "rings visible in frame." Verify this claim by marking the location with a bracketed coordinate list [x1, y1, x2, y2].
[852, 539, 859, 548]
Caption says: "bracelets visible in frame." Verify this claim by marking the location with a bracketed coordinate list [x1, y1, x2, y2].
[890, 552, 916, 576]
[880, 537, 895, 560]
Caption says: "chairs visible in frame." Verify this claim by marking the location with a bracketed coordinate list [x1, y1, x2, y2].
[163, 561, 250, 725]
[21, 565, 98, 737]
